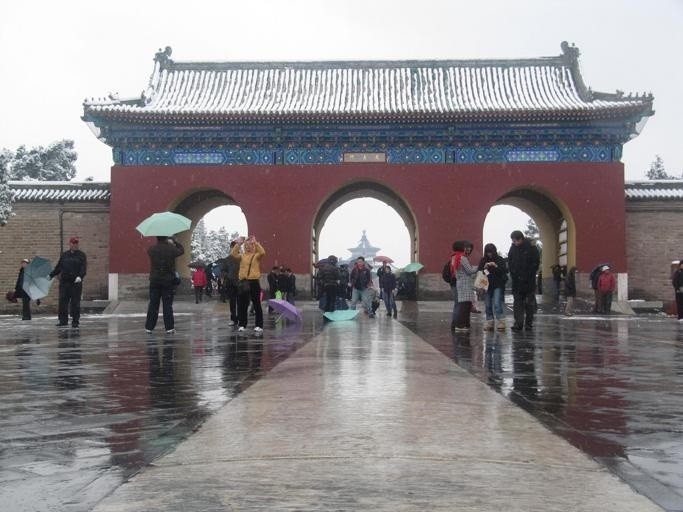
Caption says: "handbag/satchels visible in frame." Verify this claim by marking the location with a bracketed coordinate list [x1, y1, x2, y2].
[237, 279, 250, 296]
[443, 261, 450, 282]
[475, 271, 489, 290]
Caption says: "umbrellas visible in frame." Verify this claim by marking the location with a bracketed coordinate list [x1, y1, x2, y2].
[5, 289, 16, 303]
[20, 255, 54, 301]
[134, 210, 192, 238]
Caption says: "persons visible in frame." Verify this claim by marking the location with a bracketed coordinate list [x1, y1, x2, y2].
[13, 257, 40, 320]
[221, 241, 243, 327]
[395, 271, 416, 301]
[232, 235, 265, 332]
[349, 256, 375, 318]
[564, 266, 580, 316]
[379, 267, 398, 318]
[192, 267, 208, 304]
[266, 265, 297, 314]
[671, 260, 682, 322]
[377, 262, 387, 298]
[312, 255, 350, 321]
[591, 265, 614, 315]
[447, 239, 479, 331]
[508, 232, 544, 331]
[479, 243, 509, 329]
[205, 264, 231, 303]
[46, 235, 87, 327]
[364, 282, 379, 315]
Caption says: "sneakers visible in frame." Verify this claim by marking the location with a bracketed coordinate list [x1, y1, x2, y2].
[452, 319, 532, 333]
[229, 321, 263, 332]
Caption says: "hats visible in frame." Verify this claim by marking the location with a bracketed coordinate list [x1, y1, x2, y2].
[602, 266, 610, 271]
